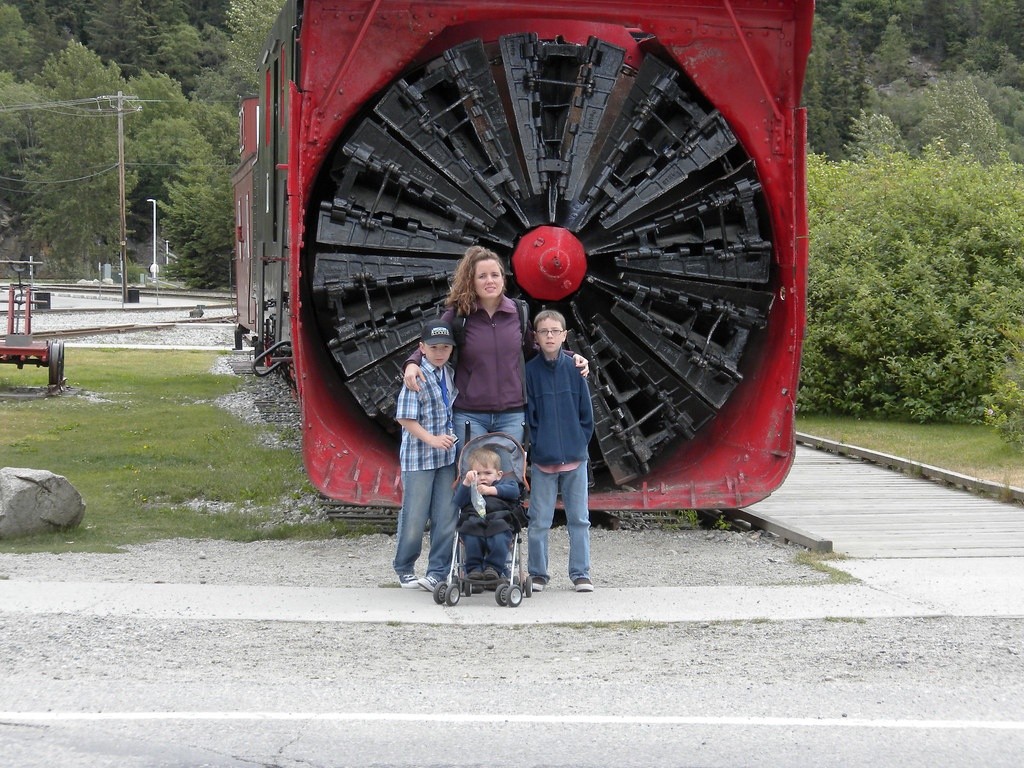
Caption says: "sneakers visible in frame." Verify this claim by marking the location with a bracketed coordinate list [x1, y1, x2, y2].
[484, 567, 499, 580]
[532, 576, 546, 591]
[399, 574, 419, 589]
[574, 576, 593, 592]
[418, 576, 440, 592]
[468, 570, 483, 579]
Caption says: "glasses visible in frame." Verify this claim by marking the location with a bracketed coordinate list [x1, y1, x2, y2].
[536, 328, 564, 336]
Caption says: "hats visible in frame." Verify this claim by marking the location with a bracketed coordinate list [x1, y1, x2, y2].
[421, 320, 457, 346]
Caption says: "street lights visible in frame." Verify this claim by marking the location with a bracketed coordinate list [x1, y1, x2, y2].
[147, 199, 159, 278]
[166, 240, 169, 281]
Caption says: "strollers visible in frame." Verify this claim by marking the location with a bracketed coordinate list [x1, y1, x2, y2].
[432, 421, 534, 608]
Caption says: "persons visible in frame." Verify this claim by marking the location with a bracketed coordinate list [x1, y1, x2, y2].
[392, 319, 458, 594]
[402, 244, 589, 463]
[453, 449, 520, 580]
[525, 309, 595, 592]
